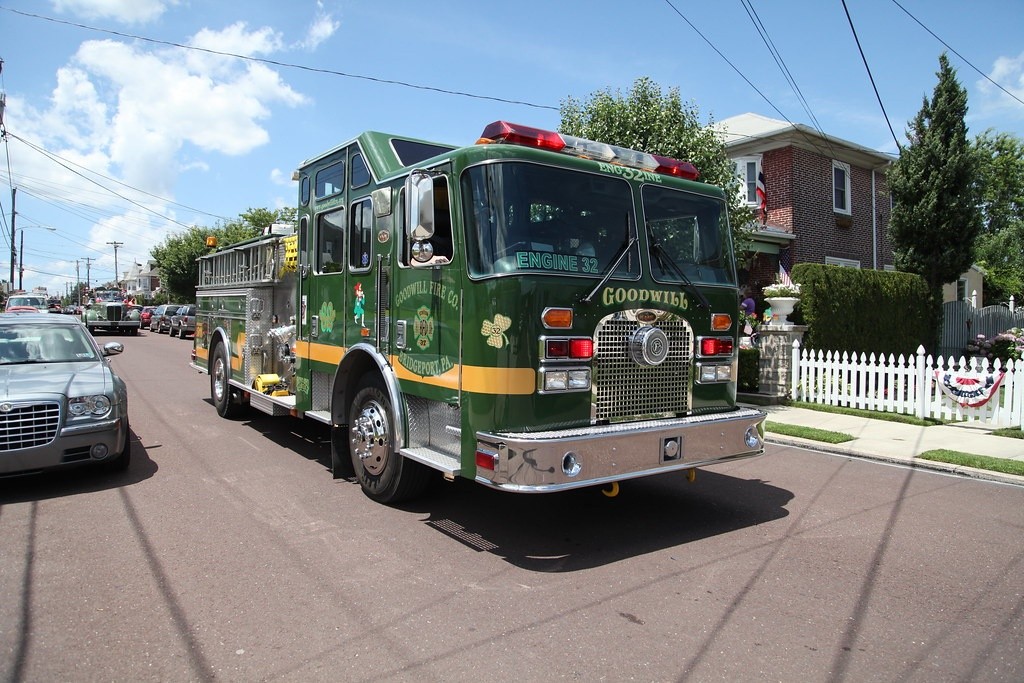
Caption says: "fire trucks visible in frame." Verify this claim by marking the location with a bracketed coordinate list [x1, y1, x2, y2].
[190, 121, 767, 506]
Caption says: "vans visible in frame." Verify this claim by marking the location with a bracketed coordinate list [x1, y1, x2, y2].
[6, 295, 62, 314]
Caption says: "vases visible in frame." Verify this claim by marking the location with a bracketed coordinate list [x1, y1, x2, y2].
[764, 297, 800, 324]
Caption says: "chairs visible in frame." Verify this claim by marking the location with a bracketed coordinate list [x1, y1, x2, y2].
[41, 333, 66, 358]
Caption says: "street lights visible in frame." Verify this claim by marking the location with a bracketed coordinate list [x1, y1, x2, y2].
[10, 227, 56, 293]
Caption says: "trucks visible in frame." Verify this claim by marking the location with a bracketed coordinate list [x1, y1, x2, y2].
[82, 287, 142, 336]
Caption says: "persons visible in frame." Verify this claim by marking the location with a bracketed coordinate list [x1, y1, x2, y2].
[739, 289, 755, 317]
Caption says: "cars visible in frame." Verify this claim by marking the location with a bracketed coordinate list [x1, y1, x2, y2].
[62, 305, 84, 314]
[129, 304, 197, 339]
[0, 306, 129, 480]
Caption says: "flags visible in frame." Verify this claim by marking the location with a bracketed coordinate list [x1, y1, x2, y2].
[756, 159, 768, 218]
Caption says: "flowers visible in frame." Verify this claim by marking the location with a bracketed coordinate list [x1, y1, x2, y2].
[762, 271, 802, 297]
